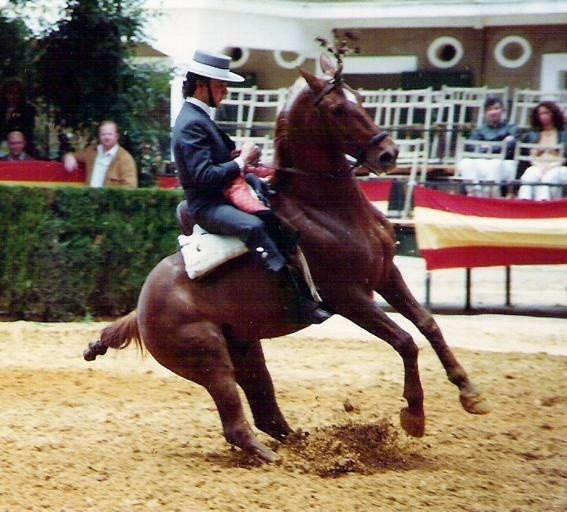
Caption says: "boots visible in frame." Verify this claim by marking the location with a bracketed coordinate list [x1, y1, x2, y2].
[279, 271, 329, 325]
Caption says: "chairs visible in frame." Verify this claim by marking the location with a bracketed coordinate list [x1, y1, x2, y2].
[210, 84, 567, 220]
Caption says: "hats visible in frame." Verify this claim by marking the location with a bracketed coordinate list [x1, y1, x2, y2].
[183, 47, 246, 83]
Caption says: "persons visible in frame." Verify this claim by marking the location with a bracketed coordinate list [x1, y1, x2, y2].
[167, 49, 332, 325]
[1, 131, 36, 161]
[62, 120, 139, 189]
[505, 100, 566, 201]
[462, 96, 519, 159]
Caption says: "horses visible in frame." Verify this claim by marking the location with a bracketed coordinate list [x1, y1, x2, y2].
[82, 50, 492, 473]
